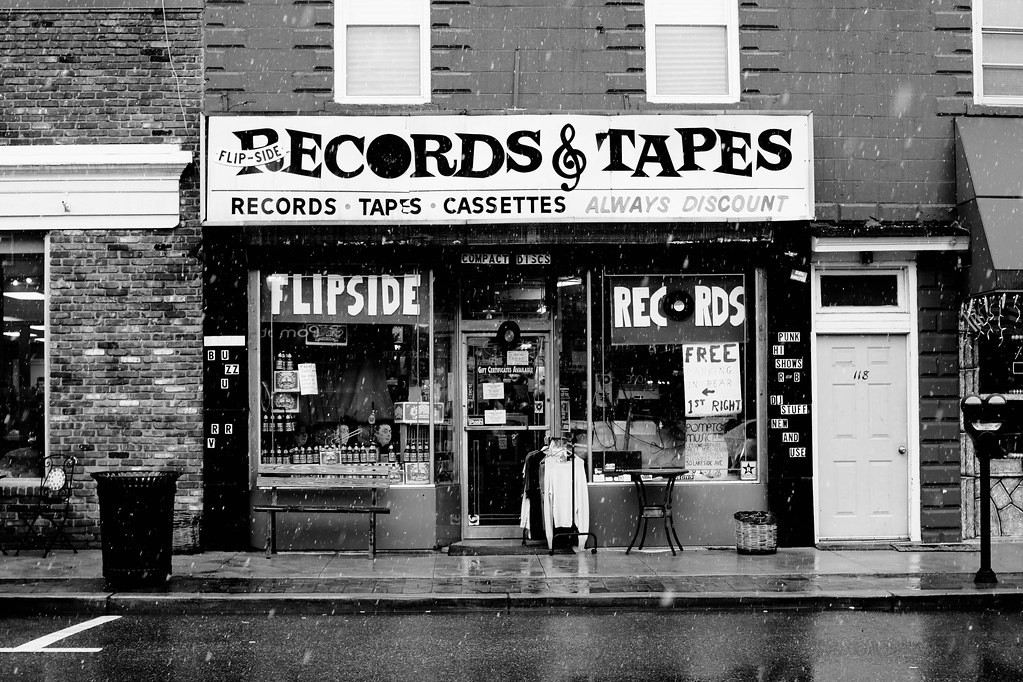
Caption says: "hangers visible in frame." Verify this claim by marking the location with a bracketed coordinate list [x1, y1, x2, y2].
[536, 436, 577, 462]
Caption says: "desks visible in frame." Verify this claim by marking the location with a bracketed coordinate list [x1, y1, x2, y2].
[609, 468, 689, 557]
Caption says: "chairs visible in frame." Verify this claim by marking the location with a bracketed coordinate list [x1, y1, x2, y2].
[12, 454, 77, 559]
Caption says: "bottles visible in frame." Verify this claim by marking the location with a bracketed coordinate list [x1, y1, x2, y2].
[261, 438, 377, 464]
[614, 466, 632, 482]
[262, 413, 297, 432]
[388, 441, 400, 463]
[403, 441, 429, 462]
[274, 348, 294, 371]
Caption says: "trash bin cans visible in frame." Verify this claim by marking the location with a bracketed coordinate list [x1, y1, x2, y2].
[90, 470, 184, 593]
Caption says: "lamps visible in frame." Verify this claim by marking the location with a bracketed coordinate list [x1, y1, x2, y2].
[860, 251, 874, 264]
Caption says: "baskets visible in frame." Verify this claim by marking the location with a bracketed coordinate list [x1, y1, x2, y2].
[733, 511, 780, 552]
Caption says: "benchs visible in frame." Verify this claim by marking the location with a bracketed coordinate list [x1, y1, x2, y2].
[253, 463, 390, 560]
